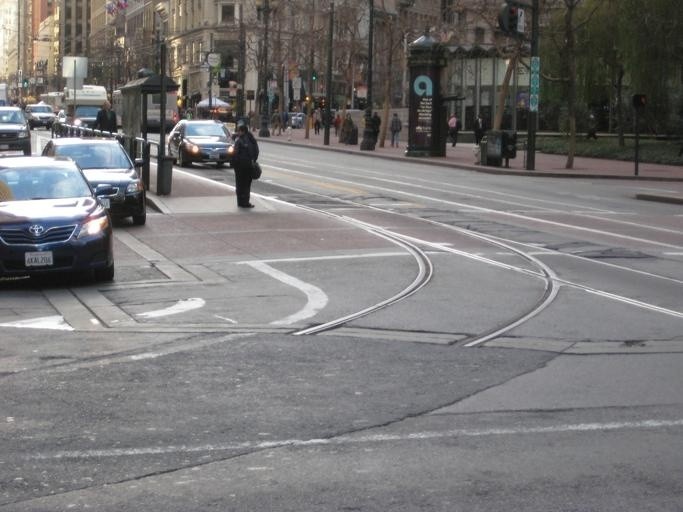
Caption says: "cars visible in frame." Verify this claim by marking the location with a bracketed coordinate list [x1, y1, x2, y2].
[168, 119, 237, 170]
[24, 103, 58, 131]
[197, 97, 232, 116]
[0, 106, 31, 157]
[357, 97, 367, 107]
[287, 112, 305, 128]
[0, 154, 116, 286]
[42, 137, 149, 227]
[57, 108, 66, 123]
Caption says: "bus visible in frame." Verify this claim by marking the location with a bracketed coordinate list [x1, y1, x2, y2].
[108, 87, 180, 129]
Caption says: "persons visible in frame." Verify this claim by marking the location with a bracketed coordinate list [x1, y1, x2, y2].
[389, 113, 402, 147]
[474, 114, 486, 146]
[184, 108, 354, 147]
[92, 101, 117, 132]
[586, 114, 599, 139]
[371, 112, 381, 143]
[448, 112, 458, 146]
[230, 126, 262, 208]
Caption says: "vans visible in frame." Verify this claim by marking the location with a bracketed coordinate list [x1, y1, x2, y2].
[64, 82, 110, 131]
[0, 82, 12, 106]
[40, 91, 64, 117]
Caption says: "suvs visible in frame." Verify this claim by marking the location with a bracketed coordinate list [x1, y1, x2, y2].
[322, 109, 337, 125]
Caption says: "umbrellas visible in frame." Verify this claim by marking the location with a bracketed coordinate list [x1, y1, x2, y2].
[196, 97, 232, 119]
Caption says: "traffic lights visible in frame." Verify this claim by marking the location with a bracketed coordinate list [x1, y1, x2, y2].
[634, 94, 647, 108]
[304, 97, 310, 101]
[313, 71, 316, 80]
[321, 97, 325, 107]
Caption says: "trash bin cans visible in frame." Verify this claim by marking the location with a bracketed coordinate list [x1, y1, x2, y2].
[478, 130, 505, 166]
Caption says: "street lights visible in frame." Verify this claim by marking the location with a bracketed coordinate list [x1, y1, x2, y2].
[319, 2, 335, 144]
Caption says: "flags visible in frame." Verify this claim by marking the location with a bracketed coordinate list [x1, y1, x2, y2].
[107, 2, 116, 16]
[114, 0, 129, 9]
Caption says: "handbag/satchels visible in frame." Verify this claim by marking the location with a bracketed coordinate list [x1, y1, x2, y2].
[250, 161, 260, 179]
